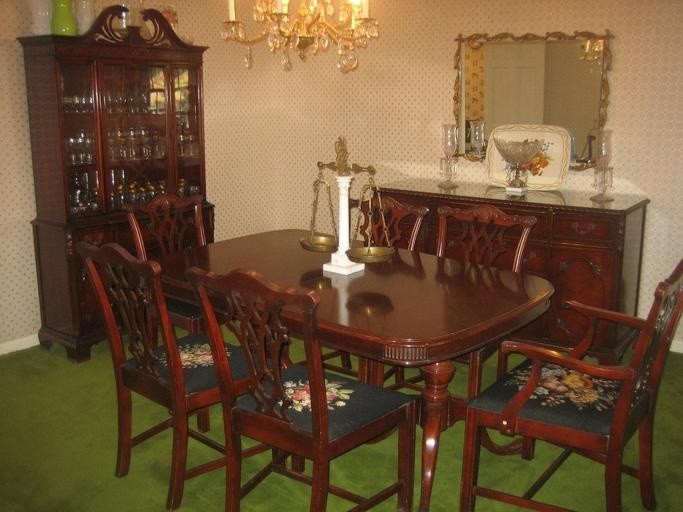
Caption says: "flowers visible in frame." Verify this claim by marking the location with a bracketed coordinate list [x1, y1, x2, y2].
[161, 9, 178, 25]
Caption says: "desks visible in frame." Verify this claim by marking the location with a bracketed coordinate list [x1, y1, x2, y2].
[146, 224, 559, 510]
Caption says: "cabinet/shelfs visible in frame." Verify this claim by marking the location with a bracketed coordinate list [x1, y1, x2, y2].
[14, 4, 217, 364]
[365, 178, 652, 344]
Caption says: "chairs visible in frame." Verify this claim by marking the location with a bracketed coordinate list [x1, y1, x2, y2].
[321, 191, 429, 392]
[180, 267, 421, 512]
[72, 239, 284, 510]
[459, 259, 682, 512]
[370, 202, 538, 434]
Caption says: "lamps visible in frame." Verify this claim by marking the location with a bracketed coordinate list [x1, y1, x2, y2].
[467, 121, 486, 155]
[214, 1, 383, 74]
[578, 38, 602, 74]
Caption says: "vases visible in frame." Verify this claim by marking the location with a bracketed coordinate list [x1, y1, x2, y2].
[50, 0, 77, 35]
[74, 0, 96, 36]
[29, 0, 52, 35]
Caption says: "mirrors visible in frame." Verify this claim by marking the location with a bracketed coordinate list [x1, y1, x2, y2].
[446, 28, 614, 171]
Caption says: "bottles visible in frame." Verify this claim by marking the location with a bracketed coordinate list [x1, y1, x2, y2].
[69, 168, 187, 211]
[63, 127, 195, 165]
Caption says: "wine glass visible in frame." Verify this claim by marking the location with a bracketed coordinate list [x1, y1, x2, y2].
[438, 123, 458, 189]
[188, 177, 201, 197]
[62, 96, 146, 113]
[590, 130, 614, 203]
[469, 120, 484, 153]
